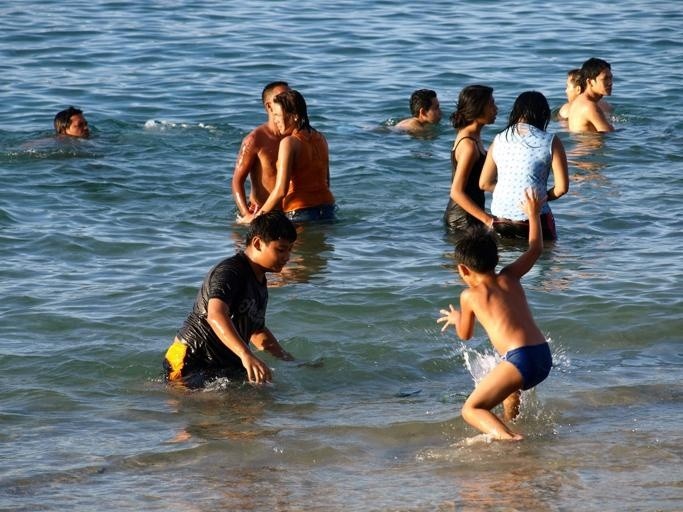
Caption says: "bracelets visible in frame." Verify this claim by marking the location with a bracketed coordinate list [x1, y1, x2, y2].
[490, 217, 494, 227]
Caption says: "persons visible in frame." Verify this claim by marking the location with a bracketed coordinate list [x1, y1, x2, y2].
[232, 82, 291, 214]
[436, 186, 553, 441]
[163, 208, 324, 391]
[396, 89, 441, 133]
[236, 90, 339, 225]
[479, 90, 568, 242]
[443, 85, 511, 235]
[557, 57, 616, 132]
[18, 106, 89, 150]
